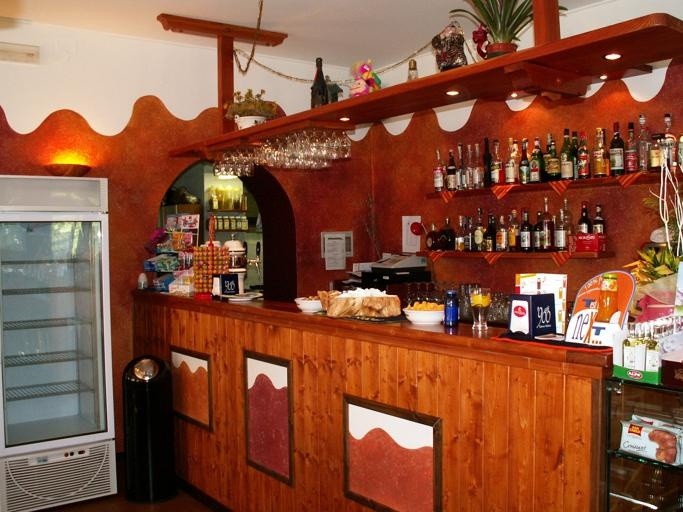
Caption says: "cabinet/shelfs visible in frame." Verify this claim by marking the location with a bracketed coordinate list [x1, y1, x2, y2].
[205, 209, 250, 233]
[599, 374, 683, 512]
[424, 169, 683, 259]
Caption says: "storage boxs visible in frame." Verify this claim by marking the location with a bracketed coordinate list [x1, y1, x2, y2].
[177, 204, 201, 231]
[161, 205, 177, 228]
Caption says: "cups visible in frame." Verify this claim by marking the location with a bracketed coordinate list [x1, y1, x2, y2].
[468, 287, 492, 331]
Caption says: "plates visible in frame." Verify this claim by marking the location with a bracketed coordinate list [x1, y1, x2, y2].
[230, 292, 264, 301]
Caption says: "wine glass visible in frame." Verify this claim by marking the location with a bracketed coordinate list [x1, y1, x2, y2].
[211, 127, 357, 179]
[400, 277, 510, 325]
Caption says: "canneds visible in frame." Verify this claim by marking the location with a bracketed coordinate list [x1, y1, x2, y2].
[214, 215, 249, 231]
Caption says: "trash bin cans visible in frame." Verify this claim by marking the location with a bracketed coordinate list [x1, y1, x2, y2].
[123, 357, 172, 503]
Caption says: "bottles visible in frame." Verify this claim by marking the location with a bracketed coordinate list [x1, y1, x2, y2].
[432, 114, 683, 193]
[425, 191, 605, 252]
[137, 272, 149, 290]
[203, 183, 254, 234]
[311, 58, 329, 110]
[406, 58, 420, 83]
[622, 312, 682, 371]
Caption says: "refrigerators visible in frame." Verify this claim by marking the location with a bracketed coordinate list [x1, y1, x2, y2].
[1, 175, 121, 512]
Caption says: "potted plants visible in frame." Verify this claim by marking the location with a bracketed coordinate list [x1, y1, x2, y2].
[447, 0, 567, 58]
[223, 87, 279, 131]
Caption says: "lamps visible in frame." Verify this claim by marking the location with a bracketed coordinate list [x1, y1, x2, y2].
[44, 163, 92, 177]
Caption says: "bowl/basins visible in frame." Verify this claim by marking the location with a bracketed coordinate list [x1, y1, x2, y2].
[293, 296, 324, 314]
